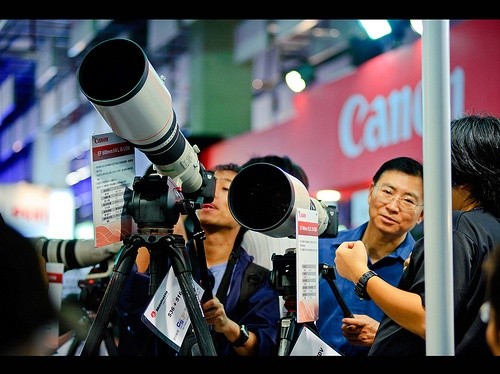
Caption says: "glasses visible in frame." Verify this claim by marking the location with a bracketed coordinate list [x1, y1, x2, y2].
[373, 185, 424, 211]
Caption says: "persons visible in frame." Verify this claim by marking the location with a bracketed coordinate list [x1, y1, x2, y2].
[0, 155, 310, 356]
[334, 115, 500, 357]
[283, 157, 424, 357]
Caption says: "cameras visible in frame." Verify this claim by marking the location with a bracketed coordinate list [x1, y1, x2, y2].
[75, 38, 216, 203]
[227, 162, 339, 238]
[30, 237, 127, 271]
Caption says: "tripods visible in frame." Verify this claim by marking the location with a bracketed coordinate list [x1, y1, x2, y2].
[269, 249, 355, 358]
[67, 174, 220, 357]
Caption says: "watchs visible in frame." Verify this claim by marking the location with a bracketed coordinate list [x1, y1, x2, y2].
[354, 271, 378, 300]
[232, 325, 249, 348]
